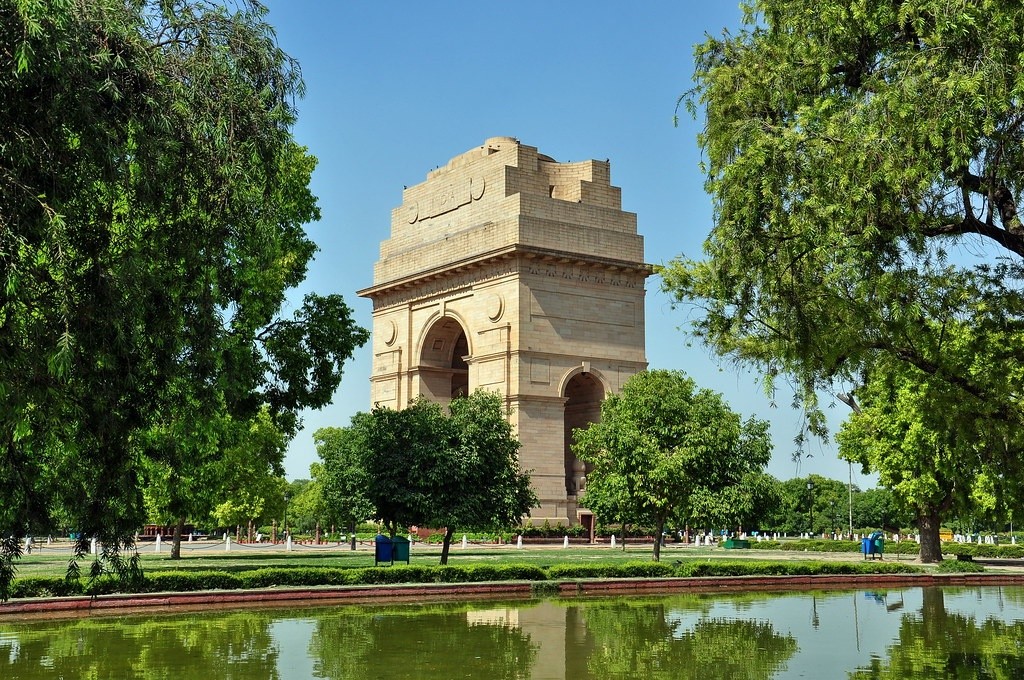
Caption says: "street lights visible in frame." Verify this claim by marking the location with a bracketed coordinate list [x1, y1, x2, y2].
[830, 499, 835, 540]
[807, 477, 814, 537]
[283, 494, 288, 537]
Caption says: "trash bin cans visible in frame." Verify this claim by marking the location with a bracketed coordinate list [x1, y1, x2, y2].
[861, 533, 885, 561]
[721, 529, 728, 535]
[70, 533, 76, 538]
[76, 533, 80, 538]
[390, 536, 410, 565]
[376, 535, 392, 566]
